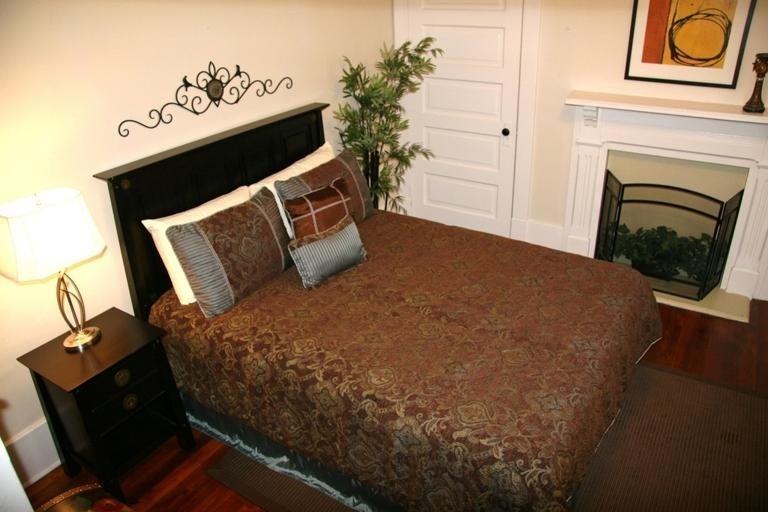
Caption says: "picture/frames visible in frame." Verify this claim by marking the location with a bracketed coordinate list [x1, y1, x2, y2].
[624, 0, 756, 89]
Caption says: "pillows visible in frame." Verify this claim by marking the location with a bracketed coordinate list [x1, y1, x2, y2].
[284, 177, 354, 240]
[287, 214, 368, 290]
[141, 185, 251, 306]
[165, 186, 294, 319]
[274, 149, 377, 229]
[249, 140, 336, 240]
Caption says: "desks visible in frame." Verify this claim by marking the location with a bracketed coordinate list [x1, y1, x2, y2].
[563, 98, 767, 303]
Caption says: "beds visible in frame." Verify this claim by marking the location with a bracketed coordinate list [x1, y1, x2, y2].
[93, 61, 664, 511]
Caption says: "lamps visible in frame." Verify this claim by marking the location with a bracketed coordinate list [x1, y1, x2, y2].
[0, 186, 108, 351]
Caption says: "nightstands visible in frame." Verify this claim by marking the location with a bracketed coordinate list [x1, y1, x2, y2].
[16, 307, 194, 501]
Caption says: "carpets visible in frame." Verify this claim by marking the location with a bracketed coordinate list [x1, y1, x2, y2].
[202, 363, 767, 512]
